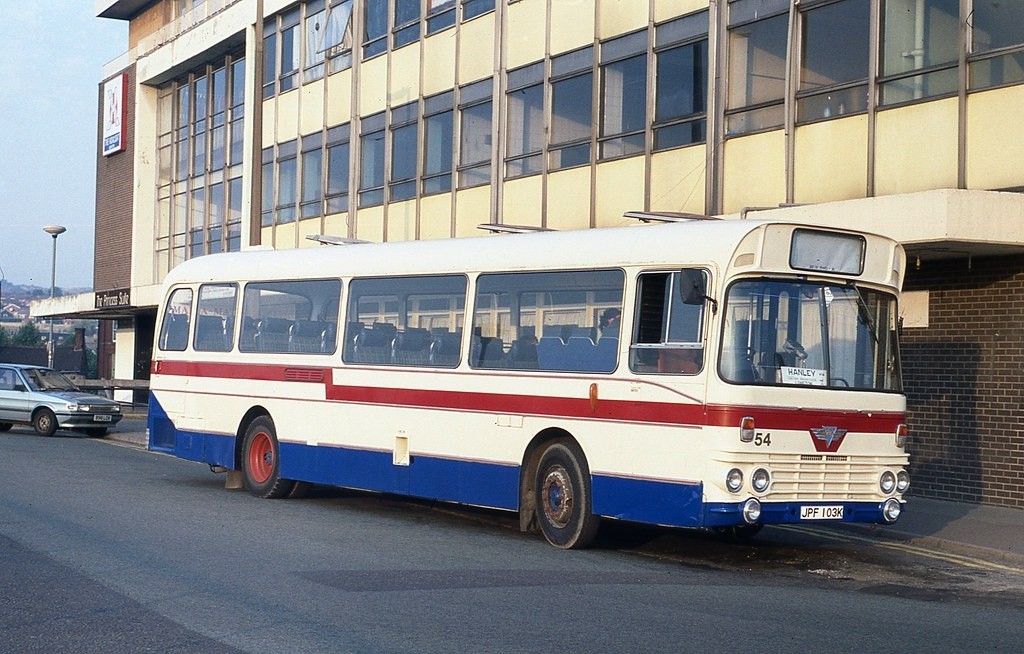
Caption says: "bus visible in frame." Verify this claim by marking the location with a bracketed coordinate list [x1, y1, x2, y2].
[141, 207, 912, 549]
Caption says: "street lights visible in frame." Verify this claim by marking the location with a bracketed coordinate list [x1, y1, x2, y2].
[43, 224, 68, 368]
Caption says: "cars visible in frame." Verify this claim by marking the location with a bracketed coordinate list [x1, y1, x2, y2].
[0, 363, 124, 438]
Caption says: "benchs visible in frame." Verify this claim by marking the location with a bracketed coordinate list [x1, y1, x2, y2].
[537, 336, 619, 372]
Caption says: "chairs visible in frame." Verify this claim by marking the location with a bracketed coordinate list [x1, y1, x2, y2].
[390, 327, 429, 364]
[223, 316, 254, 351]
[195, 315, 226, 352]
[659, 339, 699, 374]
[542, 324, 577, 342]
[320, 322, 337, 353]
[428, 332, 482, 367]
[565, 326, 598, 343]
[352, 328, 389, 364]
[288, 320, 324, 353]
[165, 314, 190, 350]
[257, 318, 290, 352]
